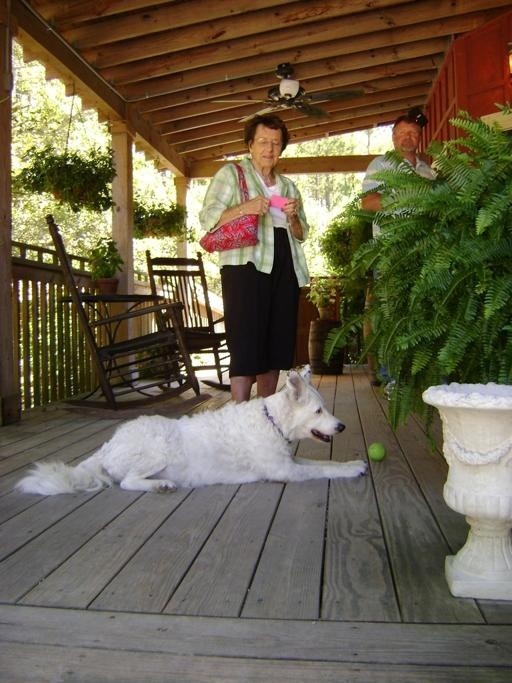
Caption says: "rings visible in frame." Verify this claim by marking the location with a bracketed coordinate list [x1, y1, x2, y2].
[295, 208, 297, 212]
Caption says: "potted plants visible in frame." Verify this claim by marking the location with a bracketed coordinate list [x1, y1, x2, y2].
[307, 270, 343, 320]
[132, 184, 199, 244]
[9, 144, 121, 215]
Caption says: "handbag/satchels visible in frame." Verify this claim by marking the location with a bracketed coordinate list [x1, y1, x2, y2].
[199, 162, 259, 253]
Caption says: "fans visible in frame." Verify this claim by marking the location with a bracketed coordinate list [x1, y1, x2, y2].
[208, 84, 366, 124]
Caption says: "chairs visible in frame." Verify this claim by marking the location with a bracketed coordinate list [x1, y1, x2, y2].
[44, 214, 231, 419]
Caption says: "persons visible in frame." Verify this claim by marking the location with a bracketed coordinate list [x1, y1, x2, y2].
[196, 111, 311, 403]
[360, 114, 437, 388]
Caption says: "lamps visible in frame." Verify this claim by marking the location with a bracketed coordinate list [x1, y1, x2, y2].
[273, 61, 300, 98]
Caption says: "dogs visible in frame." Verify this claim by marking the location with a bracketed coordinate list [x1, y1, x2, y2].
[13, 363, 369, 498]
